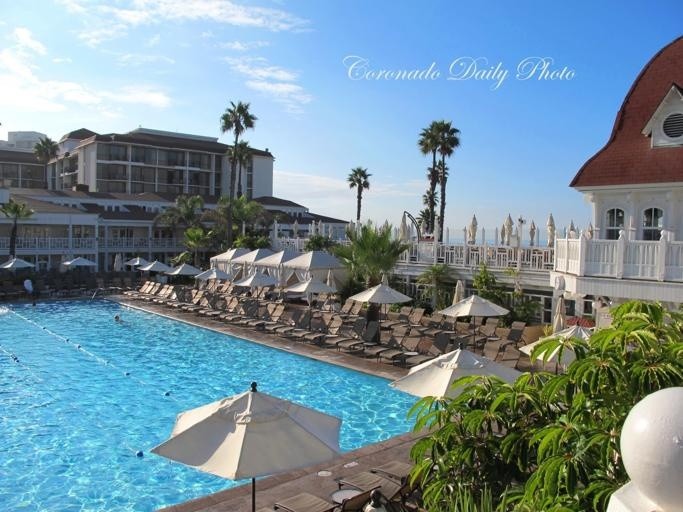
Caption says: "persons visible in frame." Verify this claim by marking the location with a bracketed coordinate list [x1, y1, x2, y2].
[24, 278, 34, 298]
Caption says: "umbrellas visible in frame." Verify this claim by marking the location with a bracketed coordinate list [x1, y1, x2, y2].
[387, 342, 525, 435]
[151, 382, 343, 511]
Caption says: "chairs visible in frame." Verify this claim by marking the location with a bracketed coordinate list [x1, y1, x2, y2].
[275, 460, 435, 512]
[0, 266, 528, 369]
